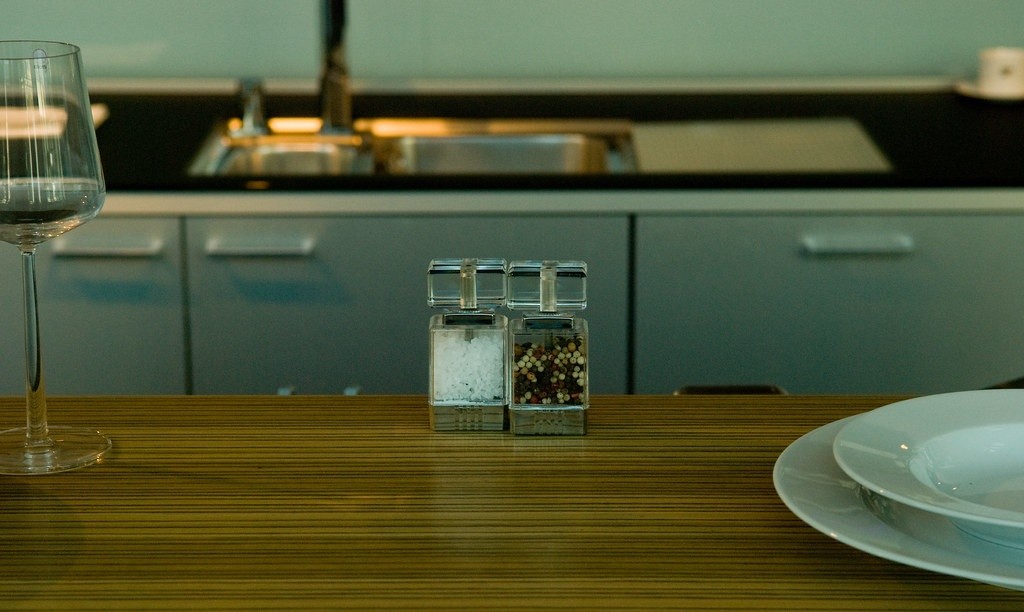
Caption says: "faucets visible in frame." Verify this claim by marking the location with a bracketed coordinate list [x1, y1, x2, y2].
[319, 0, 353, 129]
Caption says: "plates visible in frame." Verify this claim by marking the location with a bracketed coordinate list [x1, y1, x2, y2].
[772, 409, 1024, 592]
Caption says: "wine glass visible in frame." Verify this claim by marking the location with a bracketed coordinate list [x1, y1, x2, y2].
[0, 39, 112, 476]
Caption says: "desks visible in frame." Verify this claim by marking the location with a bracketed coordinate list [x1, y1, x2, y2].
[0, 396, 1024, 612]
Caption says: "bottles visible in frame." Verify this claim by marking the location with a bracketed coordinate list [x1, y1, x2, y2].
[425, 256, 508, 431]
[504, 259, 590, 437]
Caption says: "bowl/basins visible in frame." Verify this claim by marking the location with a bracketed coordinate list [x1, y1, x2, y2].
[832, 389, 1024, 550]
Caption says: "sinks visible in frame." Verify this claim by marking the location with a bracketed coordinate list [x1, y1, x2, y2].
[379, 116, 640, 179]
[187, 115, 379, 178]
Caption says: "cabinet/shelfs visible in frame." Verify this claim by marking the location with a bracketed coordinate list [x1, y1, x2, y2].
[0, 213, 1024, 396]
